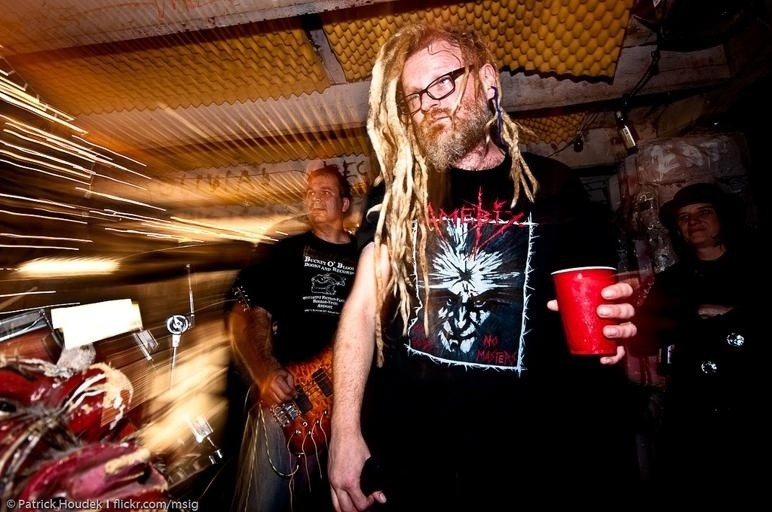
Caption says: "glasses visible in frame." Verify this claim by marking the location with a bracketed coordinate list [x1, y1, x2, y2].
[398, 65, 476, 115]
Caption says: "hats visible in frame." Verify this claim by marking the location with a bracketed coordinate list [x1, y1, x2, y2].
[658, 183, 730, 229]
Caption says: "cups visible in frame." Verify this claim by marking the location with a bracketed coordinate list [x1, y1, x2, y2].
[551, 264, 618, 358]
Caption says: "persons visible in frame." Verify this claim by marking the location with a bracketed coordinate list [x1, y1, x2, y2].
[326, 18, 645, 512]
[228, 165, 362, 510]
[633, 184, 772, 510]
